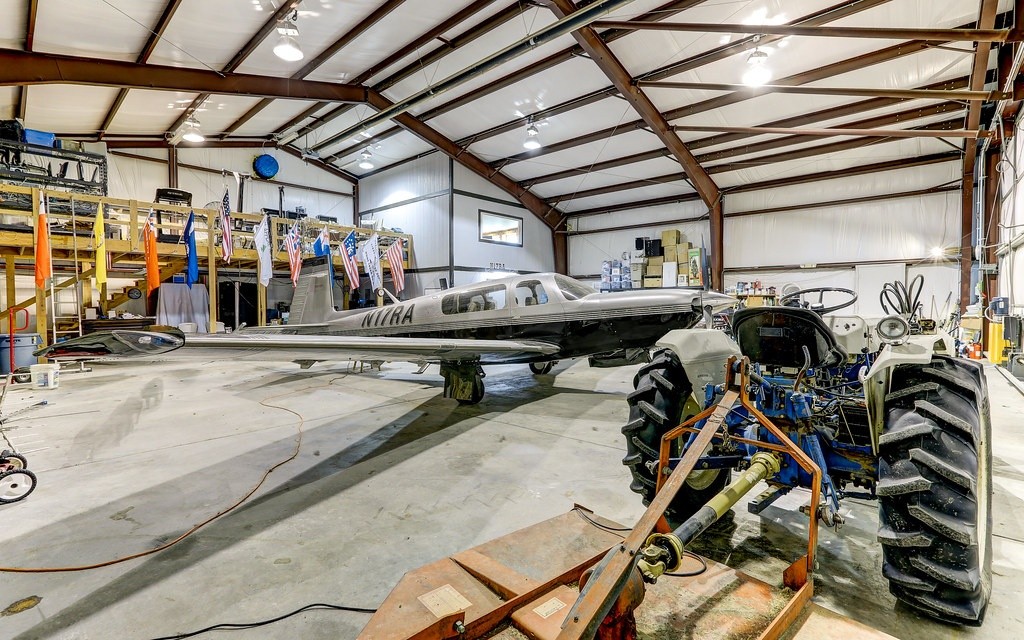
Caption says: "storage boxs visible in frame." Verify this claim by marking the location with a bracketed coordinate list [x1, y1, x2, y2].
[676, 242, 691, 263]
[661, 229, 680, 246]
[630, 251, 648, 264]
[645, 239, 664, 256]
[662, 262, 678, 287]
[55, 139, 79, 149]
[648, 256, 664, 265]
[688, 248, 706, 286]
[647, 265, 662, 275]
[80, 139, 108, 156]
[664, 245, 677, 263]
[678, 273, 690, 286]
[23, 128, 57, 146]
[0, 118, 25, 139]
[634, 237, 650, 256]
[630, 264, 646, 280]
[644, 275, 663, 288]
[631, 280, 643, 289]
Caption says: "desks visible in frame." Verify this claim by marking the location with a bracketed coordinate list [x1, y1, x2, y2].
[77, 316, 157, 337]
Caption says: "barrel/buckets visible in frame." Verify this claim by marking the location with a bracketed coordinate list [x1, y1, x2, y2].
[0, 333, 44, 379]
[30, 364, 60, 390]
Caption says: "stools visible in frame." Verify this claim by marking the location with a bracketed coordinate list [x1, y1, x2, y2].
[28, 364, 61, 390]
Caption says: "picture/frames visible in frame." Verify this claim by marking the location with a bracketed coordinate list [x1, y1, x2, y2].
[478, 209, 524, 248]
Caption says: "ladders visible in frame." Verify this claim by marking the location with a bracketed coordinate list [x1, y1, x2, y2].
[46, 192, 84, 373]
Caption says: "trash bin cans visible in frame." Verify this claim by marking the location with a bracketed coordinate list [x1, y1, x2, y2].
[0, 333, 44, 384]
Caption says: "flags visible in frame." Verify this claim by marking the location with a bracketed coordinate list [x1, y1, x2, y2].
[312, 226, 334, 290]
[284, 220, 301, 288]
[143, 208, 160, 297]
[218, 187, 233, 265]
[93, 200, 107, 294]
[362, 232, 382, 293]
[339, 229, 359, 290]
[183, 210, 198, 289]
[386, 236, 405, 297]
[35, 189, 53, 288]
[253, 214, 272, 287]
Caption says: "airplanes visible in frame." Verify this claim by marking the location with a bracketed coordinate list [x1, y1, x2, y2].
[108, 269, 741, 407]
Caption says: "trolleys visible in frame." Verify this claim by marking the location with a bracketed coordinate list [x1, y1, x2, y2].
[9, 306, 32, 382]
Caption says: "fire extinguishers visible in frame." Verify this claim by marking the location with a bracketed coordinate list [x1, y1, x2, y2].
[970, 343, 981, 359]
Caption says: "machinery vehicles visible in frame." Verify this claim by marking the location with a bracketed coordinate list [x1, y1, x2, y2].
[621, 286, 993, 631]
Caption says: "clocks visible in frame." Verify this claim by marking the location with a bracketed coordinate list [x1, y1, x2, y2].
[128, 289, 142, 300]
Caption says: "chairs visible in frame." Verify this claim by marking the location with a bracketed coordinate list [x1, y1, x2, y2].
[731, 306, 849, 375]
[525, 296, 536, 307]
[466, 295, 486, 313]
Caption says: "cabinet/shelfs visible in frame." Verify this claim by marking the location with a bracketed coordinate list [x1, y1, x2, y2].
[0, 138, 109, 238]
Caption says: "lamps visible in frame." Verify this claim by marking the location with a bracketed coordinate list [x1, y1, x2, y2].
[182, 111, 205, 143]
[273, 12, 304, 61]
[526, 115, 539, 136]
[746, 33, 768, 65]
[359, 147, 374, 169]
